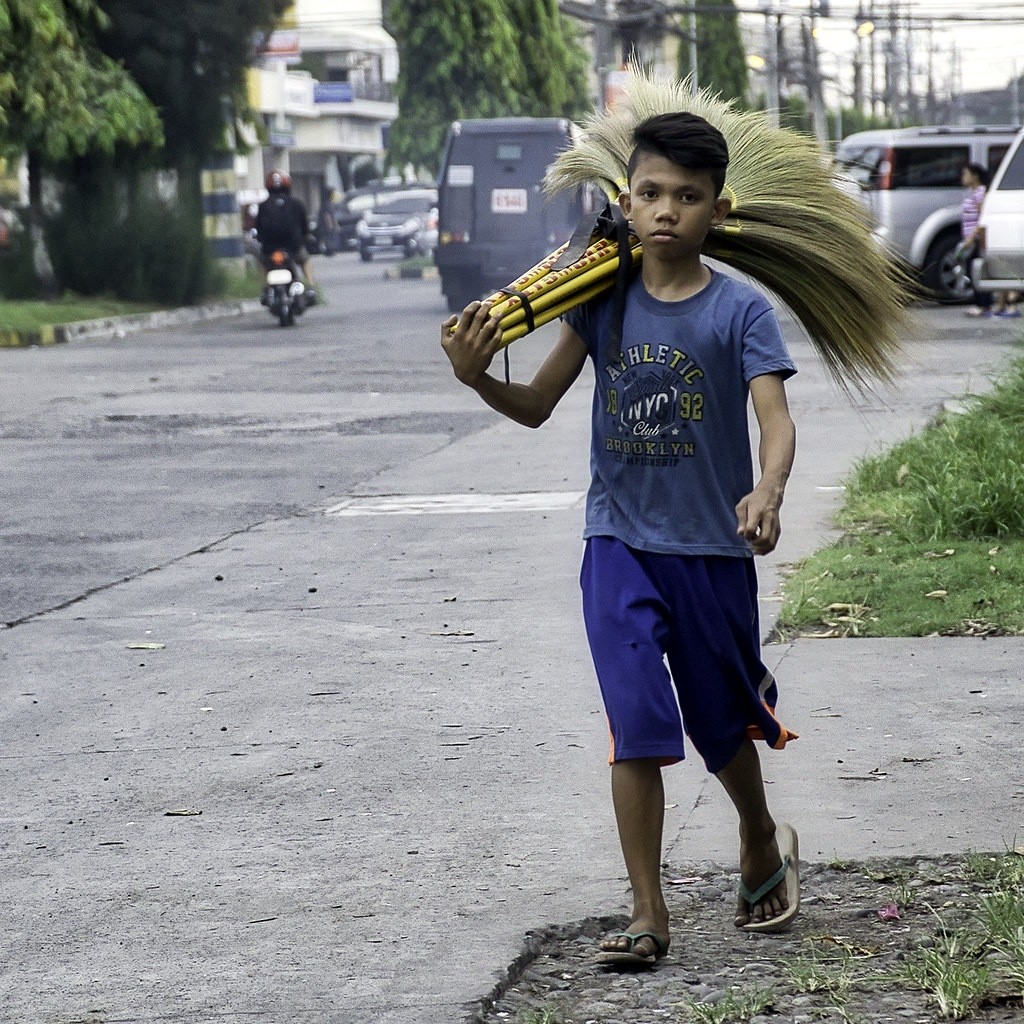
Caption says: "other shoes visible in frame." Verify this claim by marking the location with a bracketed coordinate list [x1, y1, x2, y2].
[966, 306, 991, 318]
[992, 304, 1021, 317]
[306, 287, 318, 307]
[261, 287, 275, 304]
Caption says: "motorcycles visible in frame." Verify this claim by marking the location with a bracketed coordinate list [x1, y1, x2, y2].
[247, 202, 318, 328]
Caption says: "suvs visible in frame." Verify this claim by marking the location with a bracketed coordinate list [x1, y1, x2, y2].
[968, 125, 1024, 292]
[828, 120, 1020, 306]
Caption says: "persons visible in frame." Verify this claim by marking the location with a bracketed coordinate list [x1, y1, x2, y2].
[962, 161, 991, 316]
[257, 170, 317, 306]
[319, 188, 342, 256]
[440, 111, 798, 969]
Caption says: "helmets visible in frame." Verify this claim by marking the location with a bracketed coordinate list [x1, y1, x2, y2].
[265, 170, 293, 195]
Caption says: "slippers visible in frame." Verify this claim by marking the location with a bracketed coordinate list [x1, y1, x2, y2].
[734, 822, 800, 930]
[595, 931, 668, 964]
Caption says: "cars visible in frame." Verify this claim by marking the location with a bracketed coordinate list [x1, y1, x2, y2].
[305, 180, 439, 263]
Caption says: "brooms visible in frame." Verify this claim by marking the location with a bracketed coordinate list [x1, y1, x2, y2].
[449, 43, 937, 413]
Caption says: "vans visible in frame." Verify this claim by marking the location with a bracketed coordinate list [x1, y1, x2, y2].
[428, 115, 617, 313]
